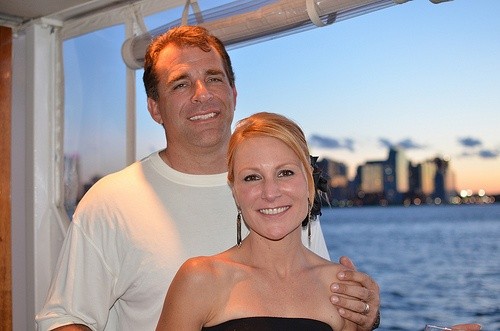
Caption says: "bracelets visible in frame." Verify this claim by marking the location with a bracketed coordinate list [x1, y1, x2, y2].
[372, 309, 381, 331]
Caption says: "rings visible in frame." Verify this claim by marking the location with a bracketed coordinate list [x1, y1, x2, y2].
[362, 302, 370, 315]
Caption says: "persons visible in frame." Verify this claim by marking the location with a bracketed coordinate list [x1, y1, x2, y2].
[34, 26, 382, 331]
[156, 112, 364, 331]
